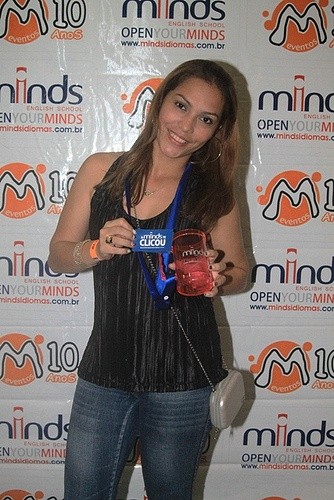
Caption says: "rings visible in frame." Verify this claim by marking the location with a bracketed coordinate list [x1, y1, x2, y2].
[106, 235, 113, 244]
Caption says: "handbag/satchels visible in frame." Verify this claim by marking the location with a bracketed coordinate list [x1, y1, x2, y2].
[209, 369, 246, 430]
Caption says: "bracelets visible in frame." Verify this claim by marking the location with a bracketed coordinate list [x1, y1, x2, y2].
[72, 239, 100, 269]
[90, 240, 104, 262]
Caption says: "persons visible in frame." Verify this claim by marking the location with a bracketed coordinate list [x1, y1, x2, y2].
[48, 58, 249, 500]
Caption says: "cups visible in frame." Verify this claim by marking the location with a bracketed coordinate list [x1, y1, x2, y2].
[172, 229, 214, 296]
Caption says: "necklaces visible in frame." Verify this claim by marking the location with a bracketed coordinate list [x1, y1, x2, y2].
[143, 177, 177, 197]
